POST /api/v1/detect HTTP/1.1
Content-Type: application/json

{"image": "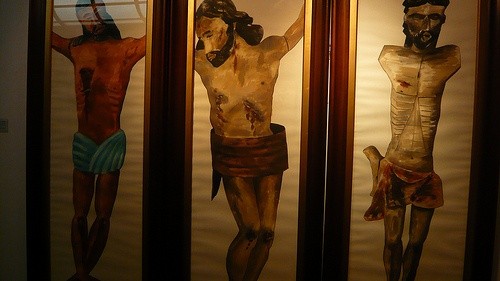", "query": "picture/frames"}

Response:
[168,0,330,280]
[27,0,173,280]
[329,0,499,281]
